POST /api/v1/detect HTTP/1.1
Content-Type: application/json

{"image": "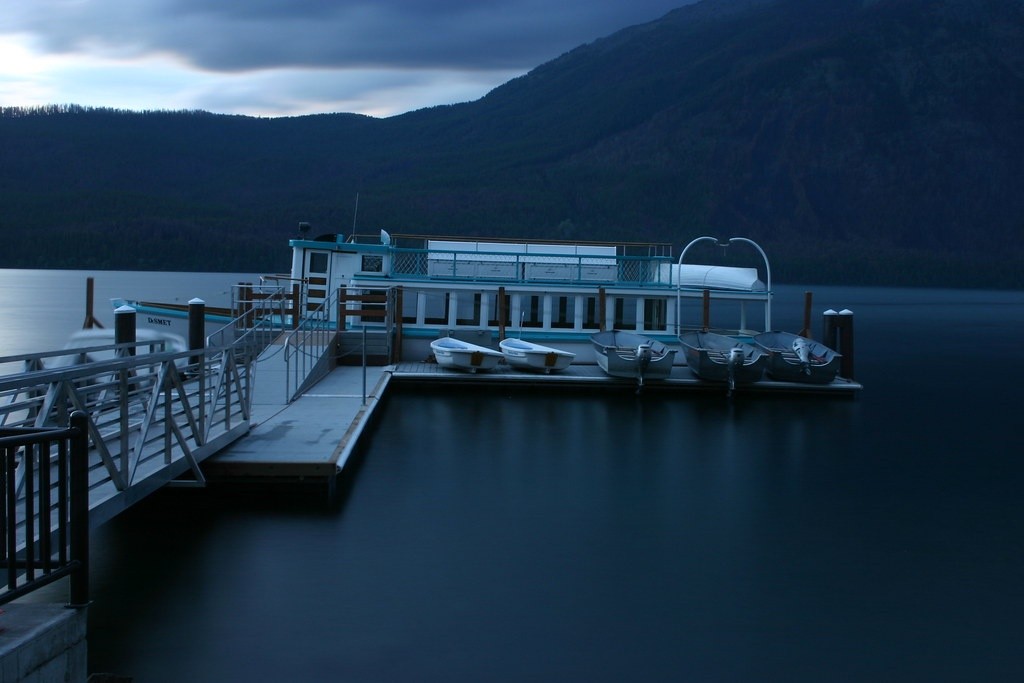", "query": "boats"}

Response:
[111,194,776,361]
[429,336,504,373]
[677,331,770,384]
[46,329,189,395]
[498,337,577,374]
[753,330,842,383]
[590,330,678,381]
